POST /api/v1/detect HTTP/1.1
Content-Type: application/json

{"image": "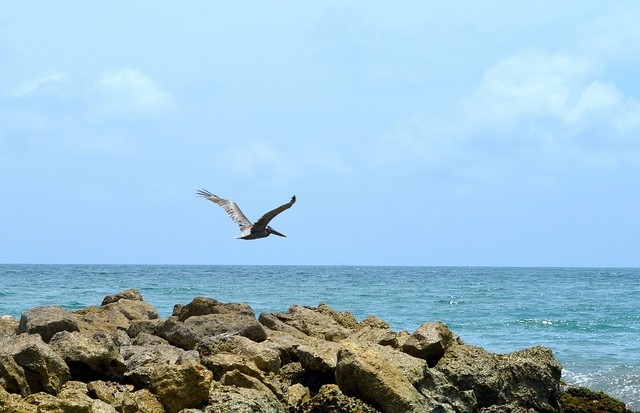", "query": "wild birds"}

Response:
[193,187,297,240]
